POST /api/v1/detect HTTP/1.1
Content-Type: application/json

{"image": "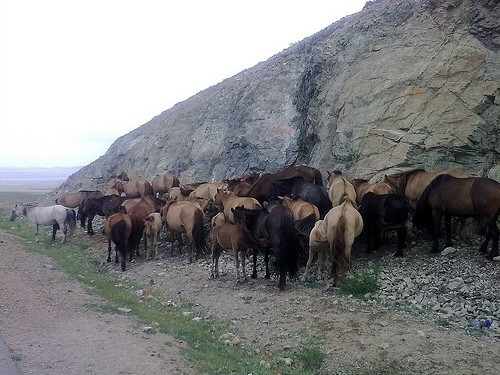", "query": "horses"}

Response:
[9,166,500,293]
